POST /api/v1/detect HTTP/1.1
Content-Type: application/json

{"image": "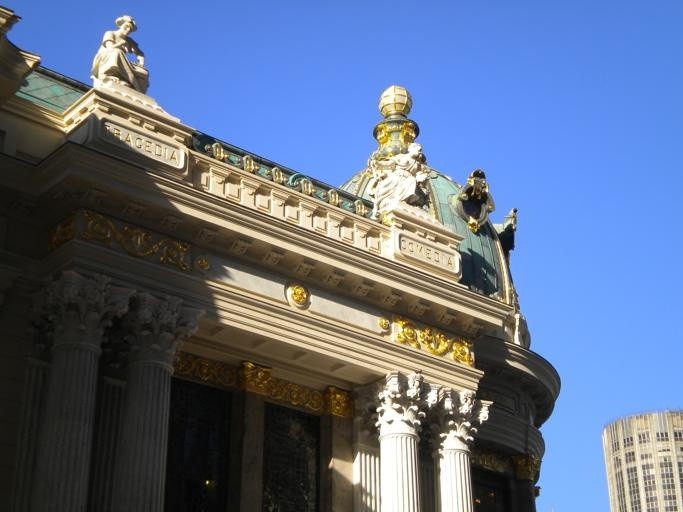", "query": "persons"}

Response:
[90,14,152,91]
[371,143,427,221]
